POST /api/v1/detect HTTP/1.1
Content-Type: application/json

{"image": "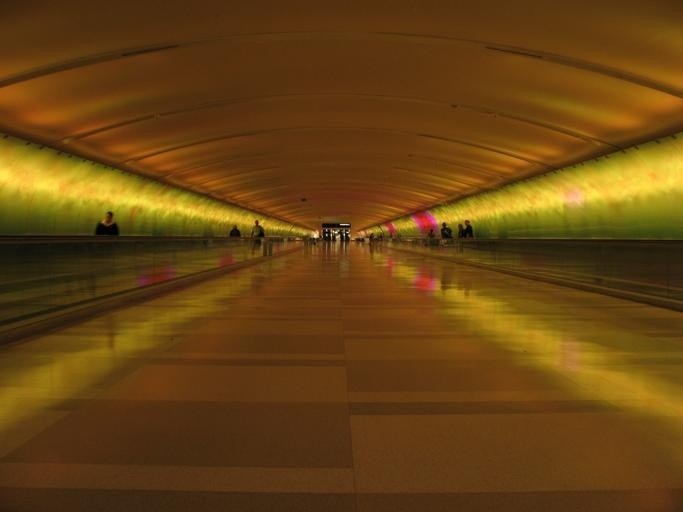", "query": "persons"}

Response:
[456,224,464,238]
[250,220,264,238]
[95,211,119,236]
[229,224,240,237]
[440,223,451,238]
[427,229,435,237]
[463,219,473,238]
